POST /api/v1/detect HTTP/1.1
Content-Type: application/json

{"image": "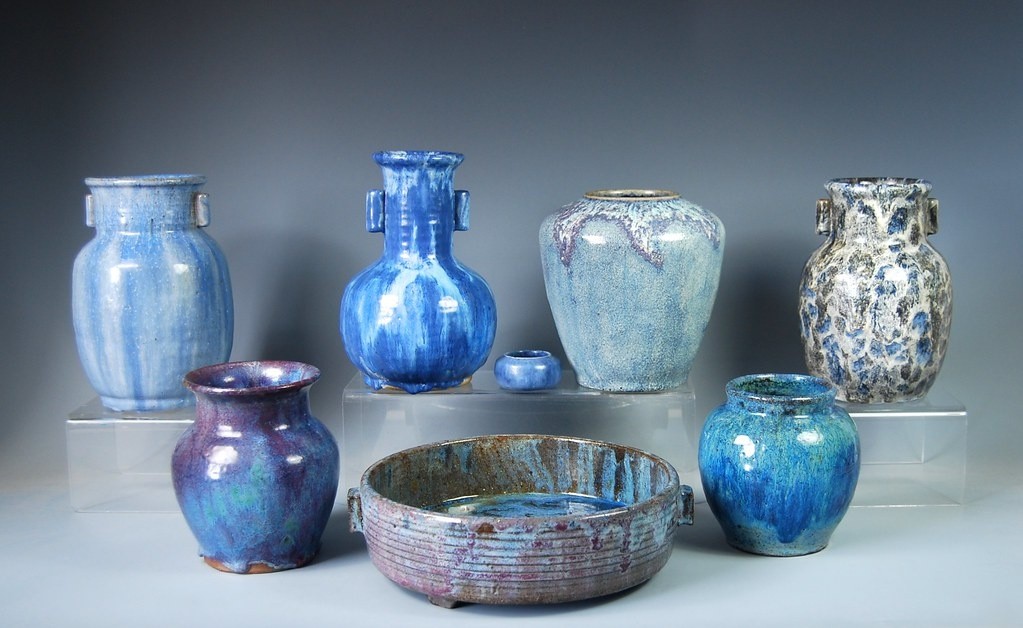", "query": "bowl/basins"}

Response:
[491,346,564,395]
[344,431,696,607]
